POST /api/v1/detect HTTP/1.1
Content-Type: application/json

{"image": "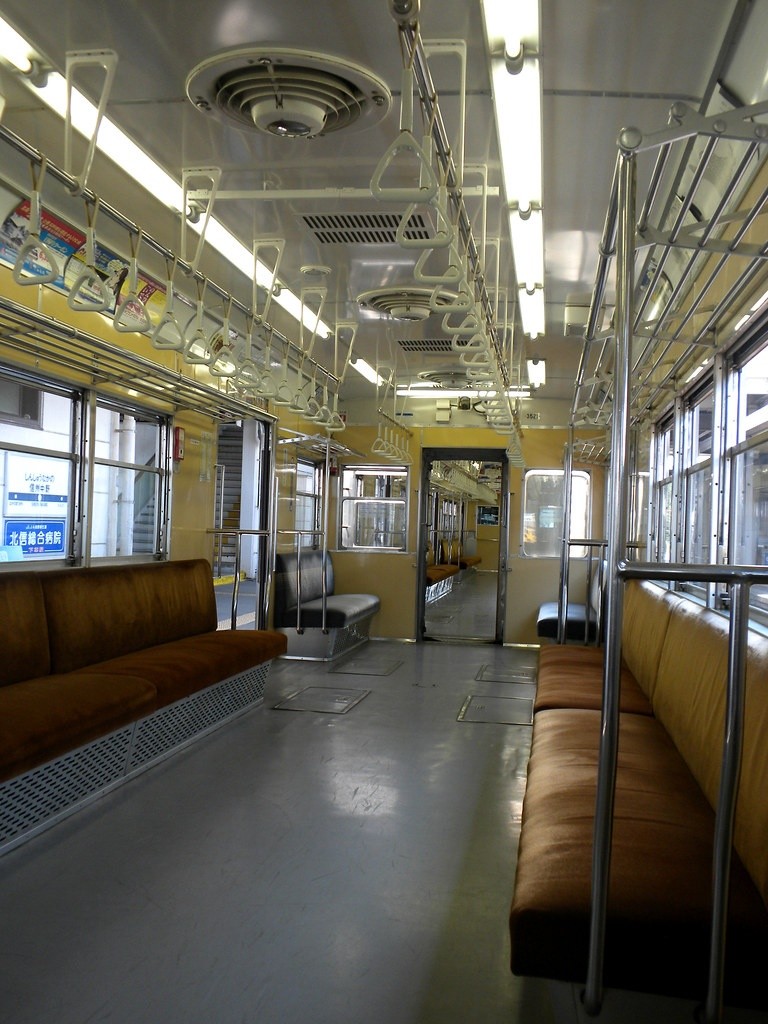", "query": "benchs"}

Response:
[427,541,460,601]
[451,540,482,573]
[0,570,156,858]
[441,539,475,584]
[425,555,447,603]
[509,560,768,1012]
[272,550,380,661]
[36,558,288,784]
